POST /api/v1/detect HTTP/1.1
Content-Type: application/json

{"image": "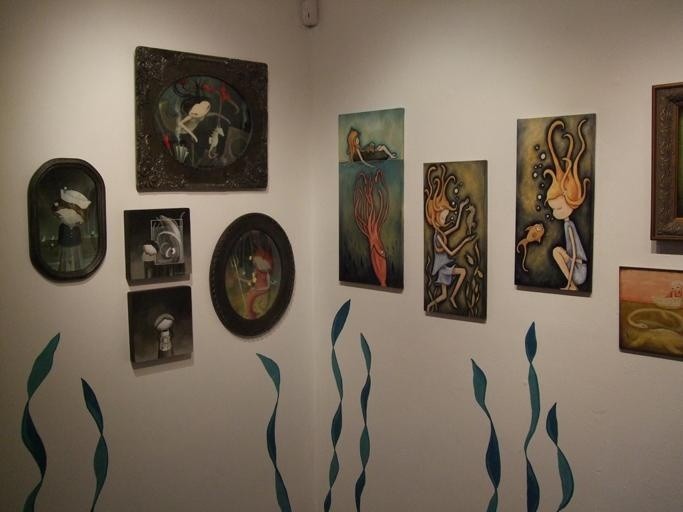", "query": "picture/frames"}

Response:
[209,213,294,339]
[123,207,193,286]
[133,46,269,191]
[28,158,107,284]
[127,286,194,364]
[618,81,683,363]
